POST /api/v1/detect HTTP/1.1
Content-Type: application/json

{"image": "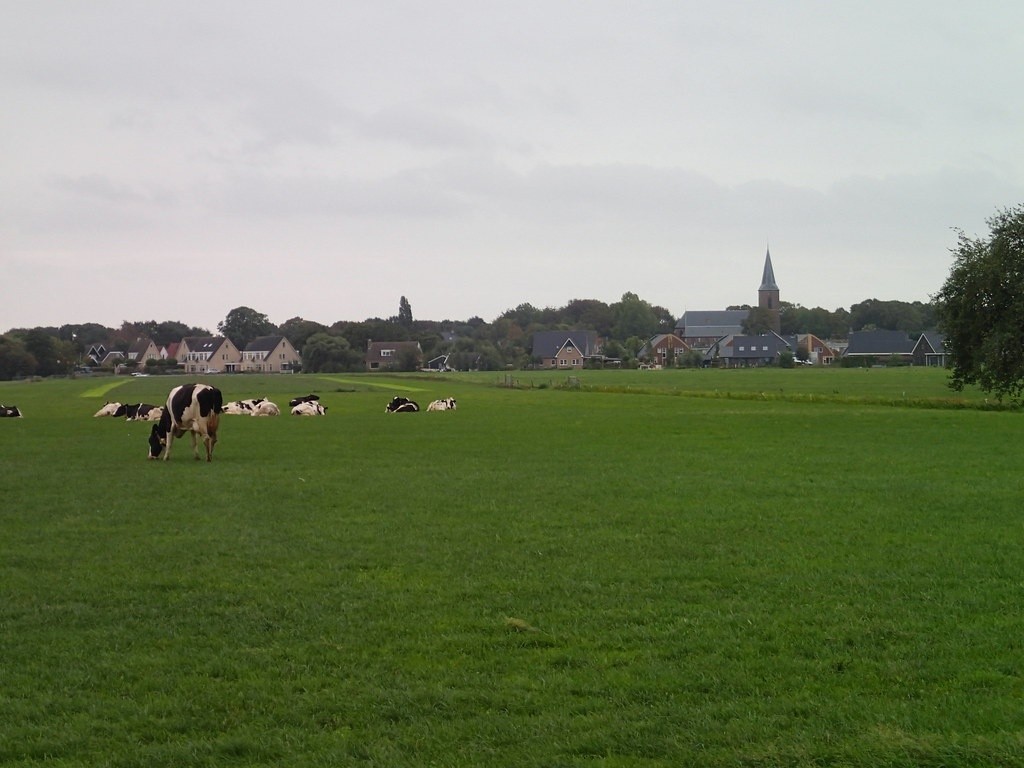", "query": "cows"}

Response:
[385,396,420,413]
[93,400,123,418]
[289,394,320,406]
[112,403,165,421]
[0,403,23,418]
[221,397,280,415]
[427,397,457,412]
[291,401,329,417]
[146,383,223,462]
